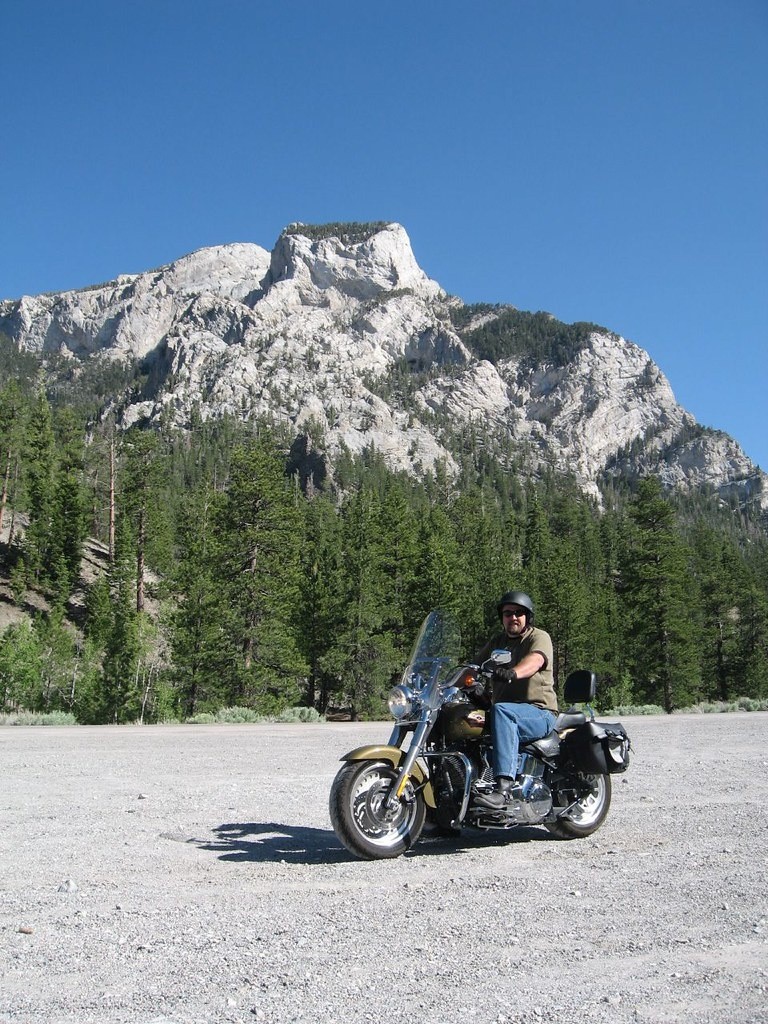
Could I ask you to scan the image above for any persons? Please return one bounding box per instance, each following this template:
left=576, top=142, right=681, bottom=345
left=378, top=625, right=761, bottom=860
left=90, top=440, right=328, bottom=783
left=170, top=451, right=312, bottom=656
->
left=472, top=591, right=559, bottom=809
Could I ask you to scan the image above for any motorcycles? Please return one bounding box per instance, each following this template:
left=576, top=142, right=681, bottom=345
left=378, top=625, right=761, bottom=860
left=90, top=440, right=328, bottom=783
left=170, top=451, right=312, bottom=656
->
left=329, top=608, right=631, bottom=859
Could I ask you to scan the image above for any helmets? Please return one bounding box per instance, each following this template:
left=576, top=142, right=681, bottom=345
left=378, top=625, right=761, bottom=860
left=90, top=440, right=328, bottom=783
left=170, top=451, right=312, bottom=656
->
left=497, top=591, right=533, bottom=613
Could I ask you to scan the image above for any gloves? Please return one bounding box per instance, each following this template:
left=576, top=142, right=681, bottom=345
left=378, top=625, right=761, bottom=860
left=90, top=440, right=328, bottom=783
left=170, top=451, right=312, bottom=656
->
left=494, top=668, right=517, bottom=682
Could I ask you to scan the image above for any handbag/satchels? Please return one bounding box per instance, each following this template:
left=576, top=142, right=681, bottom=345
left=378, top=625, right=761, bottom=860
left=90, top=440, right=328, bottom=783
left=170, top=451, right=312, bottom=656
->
left=566, top=721, right=630, bottom=776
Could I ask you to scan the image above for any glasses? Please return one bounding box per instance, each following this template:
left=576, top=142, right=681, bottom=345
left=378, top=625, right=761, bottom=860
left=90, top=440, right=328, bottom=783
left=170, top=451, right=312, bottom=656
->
left=501, top=610, right=529, bottom=617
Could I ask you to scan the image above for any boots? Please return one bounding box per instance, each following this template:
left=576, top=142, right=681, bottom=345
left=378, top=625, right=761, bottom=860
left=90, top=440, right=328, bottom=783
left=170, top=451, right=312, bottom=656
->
left=472, top=777, right=513, bottom=808
